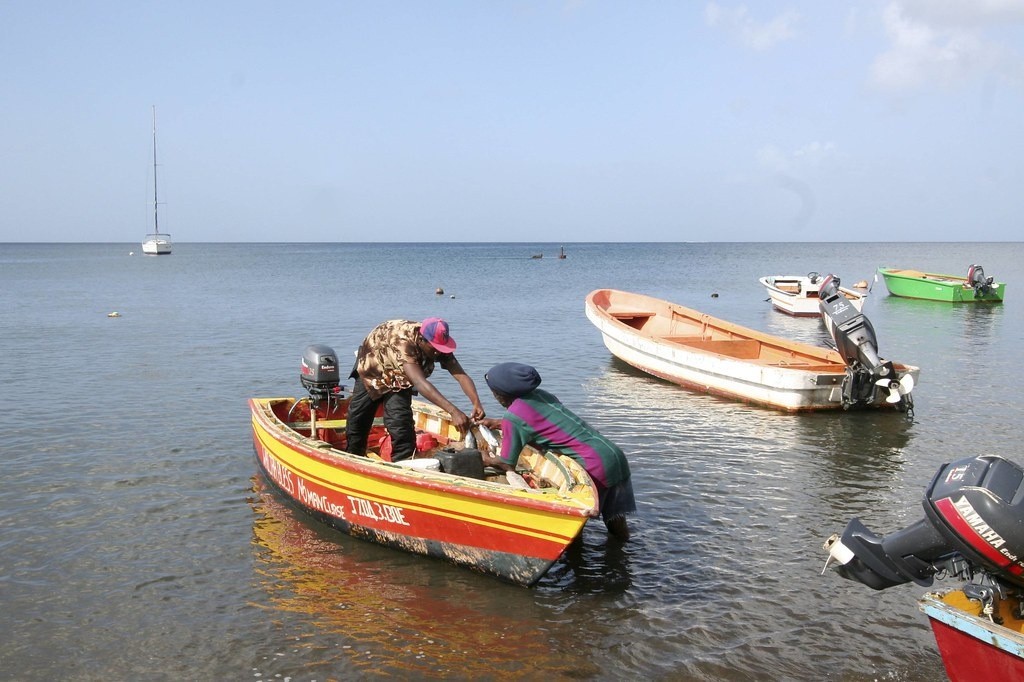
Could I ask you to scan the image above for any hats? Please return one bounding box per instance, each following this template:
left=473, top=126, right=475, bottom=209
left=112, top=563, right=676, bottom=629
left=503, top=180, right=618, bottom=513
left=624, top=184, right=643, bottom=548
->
left=420, top=317, right=457, bottom=355
left=485, top=362, right=542, bottom=394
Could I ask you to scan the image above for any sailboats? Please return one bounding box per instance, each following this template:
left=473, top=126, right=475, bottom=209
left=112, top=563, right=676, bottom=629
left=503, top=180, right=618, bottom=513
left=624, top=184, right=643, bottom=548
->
left=141, top=104, right=173, bottom=256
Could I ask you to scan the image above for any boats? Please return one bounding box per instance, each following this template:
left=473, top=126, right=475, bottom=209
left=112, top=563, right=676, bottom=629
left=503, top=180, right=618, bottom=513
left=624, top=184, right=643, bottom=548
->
left=877, top=266, right=1006, bottom=301
left=584, top=288, right=921, bottom=416
left=824, top=452, right=1024, bottom=680
left=244, top=344, right=604, bottom=587
left=761, top=274, right=868, bottom=318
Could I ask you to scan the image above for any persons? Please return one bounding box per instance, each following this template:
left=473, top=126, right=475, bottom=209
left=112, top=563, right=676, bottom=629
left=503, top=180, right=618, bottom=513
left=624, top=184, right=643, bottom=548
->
left=474, top=362, right=637, bottom=549
left=344, top=317, right=485, bottom=462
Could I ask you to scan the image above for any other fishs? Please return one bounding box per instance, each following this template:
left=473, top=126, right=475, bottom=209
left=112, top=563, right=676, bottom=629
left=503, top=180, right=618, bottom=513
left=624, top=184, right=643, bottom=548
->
left=472, top=417, right=501, bottom=447
left=463, top=426, right=478, bottom=449
